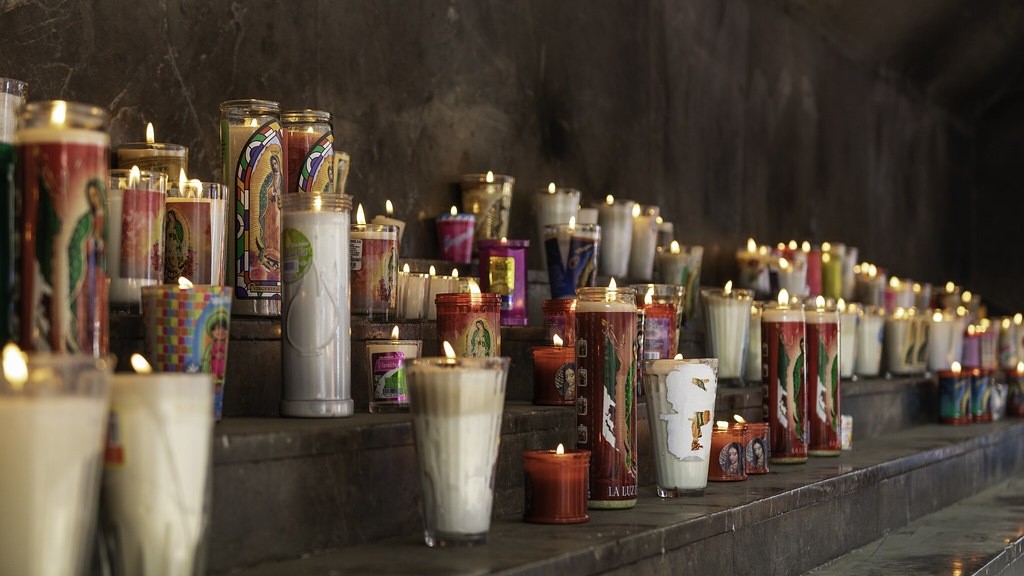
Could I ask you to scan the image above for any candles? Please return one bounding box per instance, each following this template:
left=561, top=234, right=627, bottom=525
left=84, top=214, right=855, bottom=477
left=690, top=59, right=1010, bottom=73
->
left=479, top=236, right=531, bottom=325
left=220, top=99, right=283, bottom=317
left=108, top=165, right=169, bottom=316
left=462, top=171, right=516, bottom=256
left=0, top=76, right=30, bottom=349
left=364, top=325, right=423, bottom=412
left=165, top=167, right=229, bottom=285
left=397, top=262, right=479, bottom=321
left=15, top=98, right=114, bottom=357
left=116, top=121, right=191, bottom=182
left=434, top=205, right=477, bottom=264
left=280, top=106, right=335, bottom=195
left=402, top=340, right=513, bottom=547
left=434, top=278, right=503, bottom=359
left=140, top=276, right=234, bottom=424
left=0, top=341, right=117, bottom=576
left=524, top=180, right=1024, bottom=526
left=349, top=203, right=398, bottom=323
left=279, top=191, right=354, bottom=418
left=92, top=351, right=217, bottom=576
